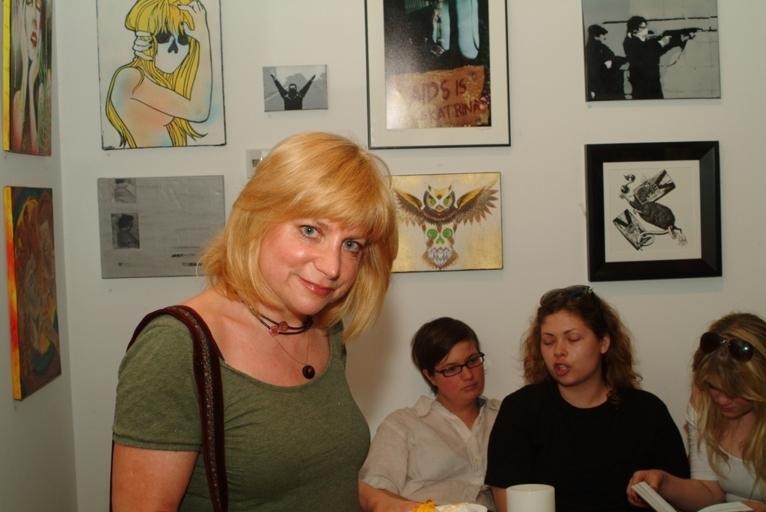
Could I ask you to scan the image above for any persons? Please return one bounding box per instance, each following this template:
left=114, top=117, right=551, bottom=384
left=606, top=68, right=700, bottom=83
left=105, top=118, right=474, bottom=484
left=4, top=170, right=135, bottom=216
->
left=108, top=132, right=398, bottom=512
left=626, top=312, right=765, bottom=512
left=622, top=14, right=697, bottom=98
left=117, top=214, right=137, bottom=248
left=269, top=73, right=314, bottom=109
left=13, top=1, right=50, bottom=156
left=114, top=179, right=135, bottom=203
left=484, top=284, right=691, bottom=511
left=585, top=24, right=628, bottom=101
left=358, top=317, right=501, bottom=512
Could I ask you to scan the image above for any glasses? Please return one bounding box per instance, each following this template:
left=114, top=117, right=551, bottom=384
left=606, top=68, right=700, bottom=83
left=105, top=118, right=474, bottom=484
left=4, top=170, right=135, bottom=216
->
left=700, top=331, right=753, bottom=362
left=540, top=286, right=601, bottom=305
left=434, top=352, right=485, bottom=377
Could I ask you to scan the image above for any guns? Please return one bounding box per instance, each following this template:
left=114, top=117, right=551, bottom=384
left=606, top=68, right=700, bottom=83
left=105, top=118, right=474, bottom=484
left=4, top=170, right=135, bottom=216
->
left=645, top=26, right=718, bottom=62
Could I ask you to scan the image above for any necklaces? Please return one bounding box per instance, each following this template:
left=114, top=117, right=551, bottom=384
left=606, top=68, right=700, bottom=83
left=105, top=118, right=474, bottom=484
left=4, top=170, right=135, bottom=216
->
left=247, top=302, right=315, bottom=380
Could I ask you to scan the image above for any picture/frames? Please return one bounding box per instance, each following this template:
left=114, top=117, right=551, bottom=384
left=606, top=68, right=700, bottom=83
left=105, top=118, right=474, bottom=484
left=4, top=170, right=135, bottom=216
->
left=584, top=140, right=722, bottom=282
left=365, top=0, right=511, bottom=150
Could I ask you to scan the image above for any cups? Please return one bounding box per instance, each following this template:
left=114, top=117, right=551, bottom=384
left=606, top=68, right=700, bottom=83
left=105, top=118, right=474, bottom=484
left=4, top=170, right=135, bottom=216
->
left=435, top=504, right=488, bottom=512
left=506, top=483, right=557, bottom=512
